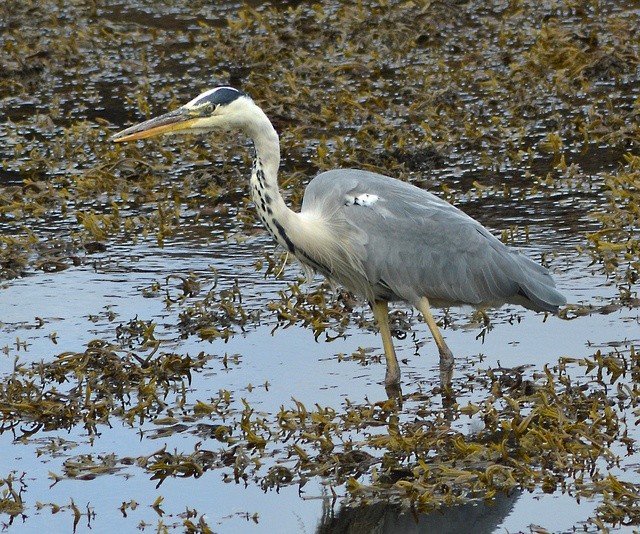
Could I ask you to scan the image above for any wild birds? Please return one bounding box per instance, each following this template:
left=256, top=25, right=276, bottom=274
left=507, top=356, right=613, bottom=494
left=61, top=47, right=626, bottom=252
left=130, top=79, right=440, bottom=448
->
left=107, top=86, right=567, bottom=387
